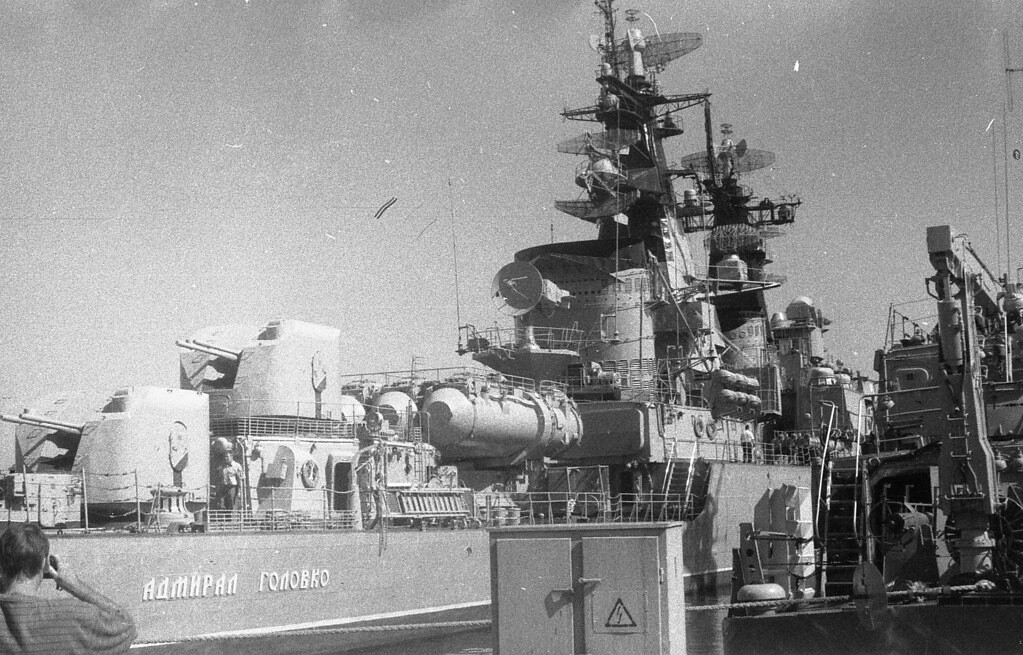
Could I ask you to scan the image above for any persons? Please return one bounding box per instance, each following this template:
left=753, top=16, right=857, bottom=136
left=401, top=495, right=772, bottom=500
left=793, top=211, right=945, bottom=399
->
left=741, top=425, right=756, bottom=463
left=0, top=523, right=138, bottom=655
left=219, top=451, right=242, bottom=522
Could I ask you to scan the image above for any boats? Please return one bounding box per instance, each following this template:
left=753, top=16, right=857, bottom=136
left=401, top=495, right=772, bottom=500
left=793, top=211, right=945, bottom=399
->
left=722, top=226, right=1023, bottom=655
left=0, top=0, right=875, bottom=651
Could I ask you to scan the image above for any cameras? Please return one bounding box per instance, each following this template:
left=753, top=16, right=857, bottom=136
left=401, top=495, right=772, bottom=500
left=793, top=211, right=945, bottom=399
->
left=43, top=554, right=57, bottom=579
